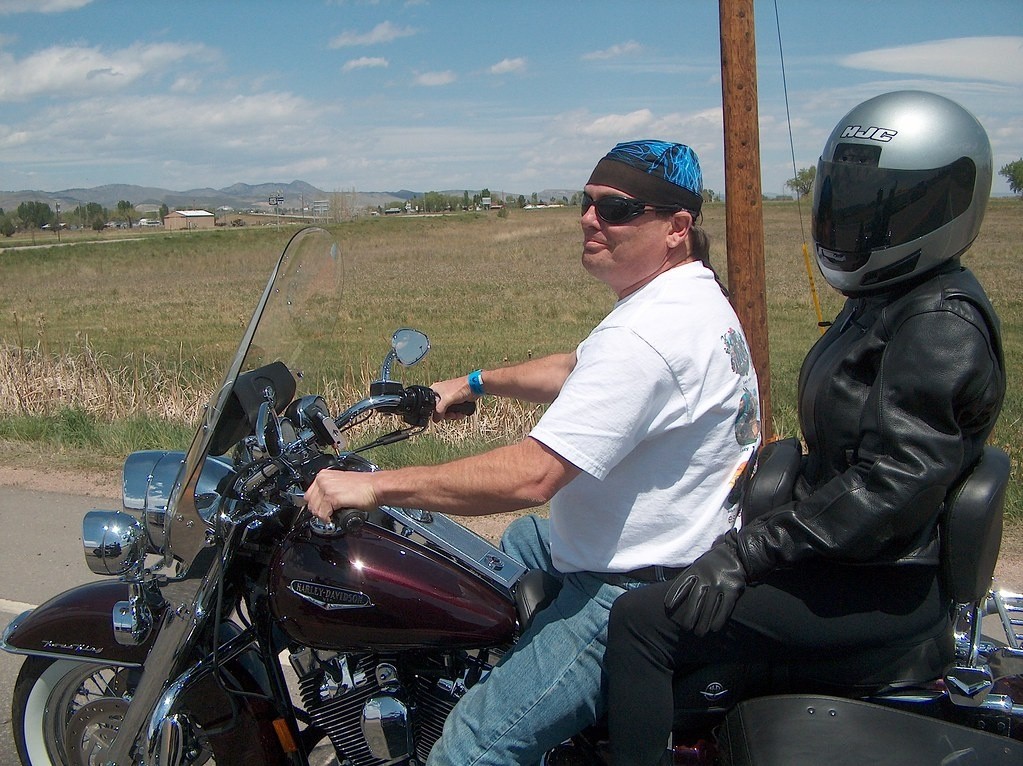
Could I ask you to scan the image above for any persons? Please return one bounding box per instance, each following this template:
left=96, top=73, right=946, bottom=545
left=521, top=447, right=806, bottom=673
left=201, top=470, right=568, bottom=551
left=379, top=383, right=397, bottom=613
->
left=302, top=139, right=763, bottom=766
left=600, top=90, right=1008, bottom=766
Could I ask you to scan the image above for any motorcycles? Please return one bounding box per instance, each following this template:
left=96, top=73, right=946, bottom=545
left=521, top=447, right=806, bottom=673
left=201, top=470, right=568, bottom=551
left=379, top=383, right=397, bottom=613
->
left=0, top=223, right=1023, bottom=766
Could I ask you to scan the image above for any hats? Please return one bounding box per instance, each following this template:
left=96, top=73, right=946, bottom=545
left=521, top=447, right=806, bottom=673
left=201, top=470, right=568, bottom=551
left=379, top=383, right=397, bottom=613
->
left=586, top=139, right=704, bottom=220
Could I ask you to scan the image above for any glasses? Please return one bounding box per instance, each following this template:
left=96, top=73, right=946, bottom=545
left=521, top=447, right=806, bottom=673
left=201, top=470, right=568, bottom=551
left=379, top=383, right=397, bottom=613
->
left=580, top=192, right=686, bottom=223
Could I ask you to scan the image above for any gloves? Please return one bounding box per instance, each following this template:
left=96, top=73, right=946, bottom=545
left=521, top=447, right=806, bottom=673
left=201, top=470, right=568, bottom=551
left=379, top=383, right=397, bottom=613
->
left=664, top=528, right=747, bottom=635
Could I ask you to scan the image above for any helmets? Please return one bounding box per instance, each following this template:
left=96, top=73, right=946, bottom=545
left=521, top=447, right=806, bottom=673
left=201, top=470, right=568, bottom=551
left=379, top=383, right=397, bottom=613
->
left=810, top=90, right=992, bottom=297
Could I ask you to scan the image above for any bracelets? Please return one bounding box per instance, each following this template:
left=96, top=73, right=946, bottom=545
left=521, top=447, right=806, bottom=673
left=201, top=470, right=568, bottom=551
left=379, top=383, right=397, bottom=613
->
left=468, top=369, right=485, bottom=396
